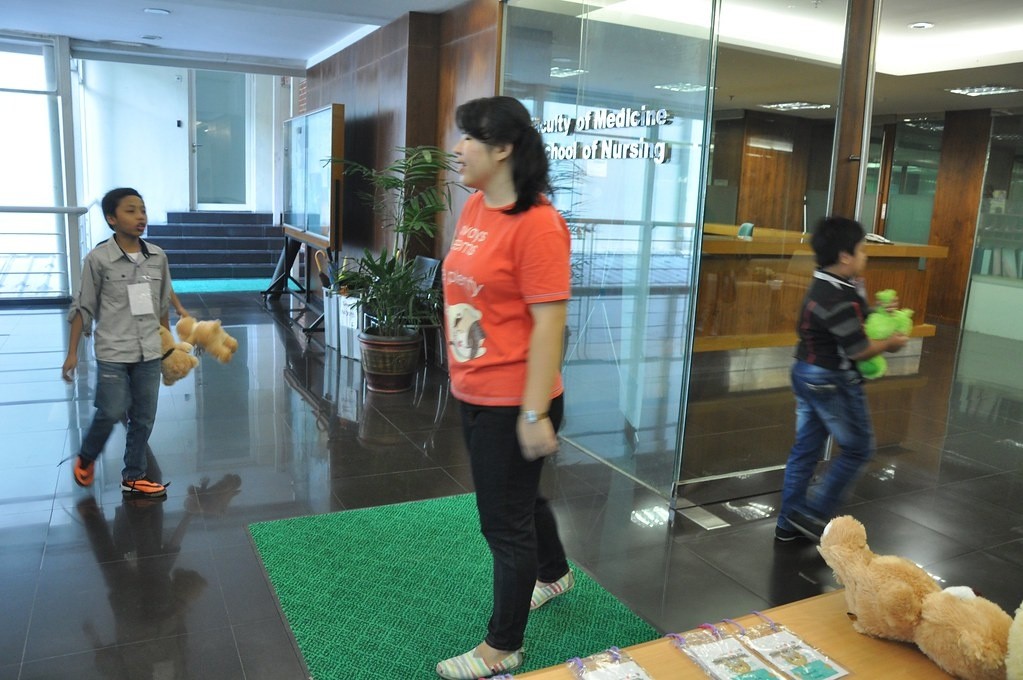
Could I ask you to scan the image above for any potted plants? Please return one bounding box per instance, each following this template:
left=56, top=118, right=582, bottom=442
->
left=320, top=144, right=473, bottom=392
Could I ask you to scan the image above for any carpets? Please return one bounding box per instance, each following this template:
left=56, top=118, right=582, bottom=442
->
left=249, top=492, right=664, bottom=680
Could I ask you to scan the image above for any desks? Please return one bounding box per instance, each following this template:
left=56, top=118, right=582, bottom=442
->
left=489, top=588, right=958, bottom=680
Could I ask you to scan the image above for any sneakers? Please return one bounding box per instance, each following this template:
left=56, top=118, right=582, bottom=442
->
left=121, top=477, right=166, bottom=497
left=75, top=455, right=94, bottom=486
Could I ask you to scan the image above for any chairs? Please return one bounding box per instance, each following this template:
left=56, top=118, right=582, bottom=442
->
left=362, top=255, right=444, bottom=365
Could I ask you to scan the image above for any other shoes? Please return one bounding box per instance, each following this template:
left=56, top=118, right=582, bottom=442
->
left=774, top=526, right=807, bottom=540
left=529, top=568, right=575, bottom=610
left=786, top=511, right=824, bottom=542
left=437, top=646, right=525, bottom=680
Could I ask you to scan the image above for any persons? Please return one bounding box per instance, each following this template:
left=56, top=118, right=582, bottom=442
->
left=61, top=187, right=189, bottom=499
left=772, top=215, right=909, bottom=541
left=435, top=95, right=580, bottom=680
left=78, top=496, right=195, bottom=680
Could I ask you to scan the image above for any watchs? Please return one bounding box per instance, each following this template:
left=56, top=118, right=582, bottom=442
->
left=520, top=409, right=548, bottom=423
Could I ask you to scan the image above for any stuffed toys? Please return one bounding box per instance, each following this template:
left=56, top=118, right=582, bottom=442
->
left=184, top=474, right=243, bottom=515
left=815, top=514, right=1023, bottom=680
left=173, top=566, right=207, bottom=601
left=158, top=323, right=199, bottom=387
left=857, top=289, right=914, bottom=378
left=175, top=317, right=238, bottom=364
